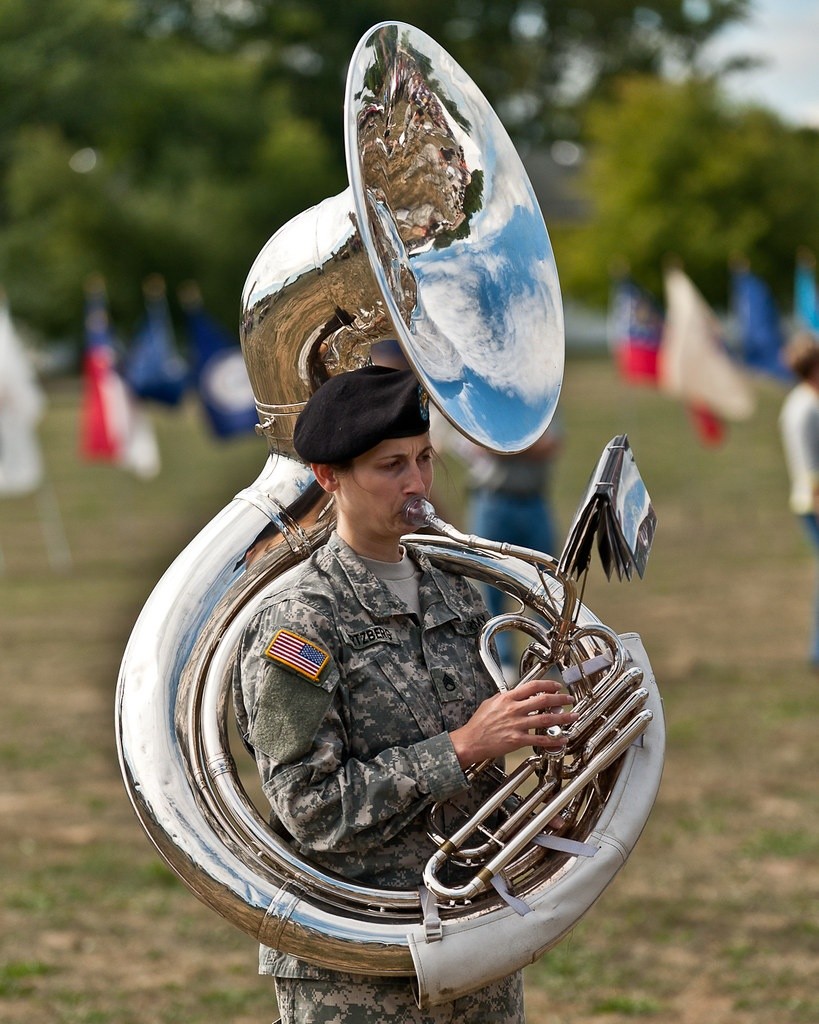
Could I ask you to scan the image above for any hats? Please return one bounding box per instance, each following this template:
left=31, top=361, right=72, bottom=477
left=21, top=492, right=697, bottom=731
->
left=294, top=365, right=430, bottom=466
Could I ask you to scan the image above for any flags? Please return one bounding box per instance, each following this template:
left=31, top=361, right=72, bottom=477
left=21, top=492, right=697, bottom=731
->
left=0, top=283, right=229, bottom=498
left=609, top=263, right=819, bottom=444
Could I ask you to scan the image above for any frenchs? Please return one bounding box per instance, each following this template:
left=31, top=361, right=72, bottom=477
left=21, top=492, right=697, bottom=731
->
left=116, top=16, right=655, bottom=977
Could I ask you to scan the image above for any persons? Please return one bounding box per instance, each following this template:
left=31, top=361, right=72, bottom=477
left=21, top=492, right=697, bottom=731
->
left=459, top=410, right=562, bottom=685
left=234, top=364, right=580, bottom=1024
left=780, top=329, right=818, bottom=667
